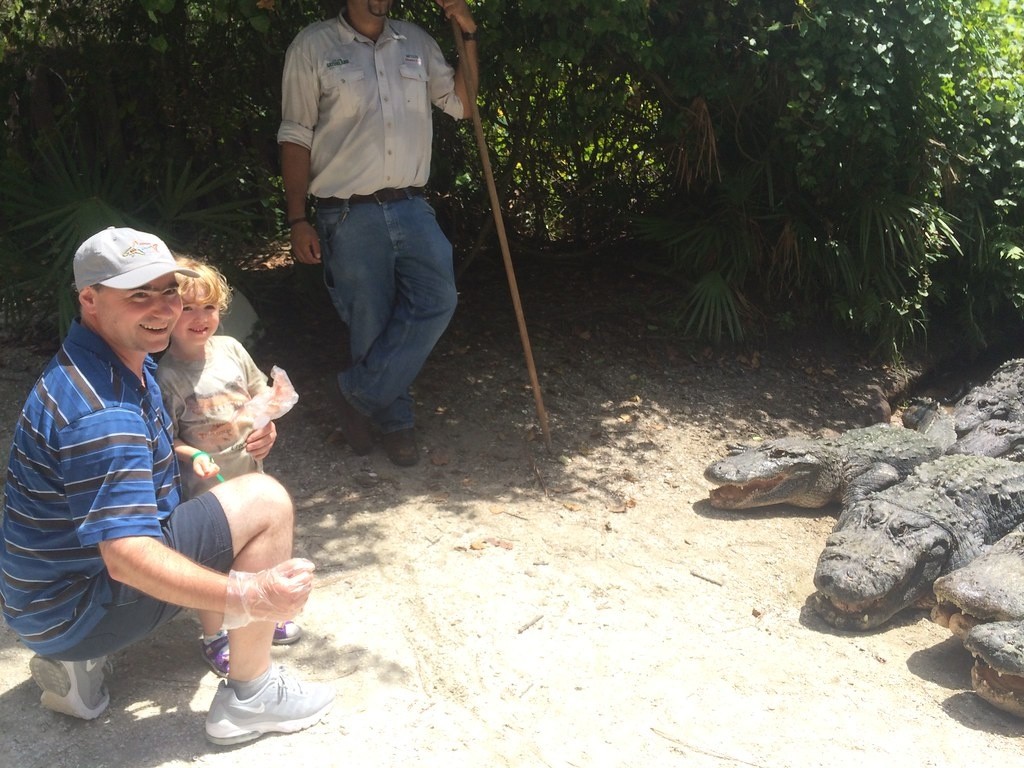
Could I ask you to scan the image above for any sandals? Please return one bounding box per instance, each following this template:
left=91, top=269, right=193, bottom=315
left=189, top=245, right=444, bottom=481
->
left=201, top=636, right=230, bottom=678
left=273, top=621, right=303, bottom=643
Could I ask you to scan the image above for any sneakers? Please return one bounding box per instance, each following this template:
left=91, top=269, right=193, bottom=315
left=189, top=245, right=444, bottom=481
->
left=205, top=664, right=336, bottom=745
left=29, top=653, right=113, bottom=721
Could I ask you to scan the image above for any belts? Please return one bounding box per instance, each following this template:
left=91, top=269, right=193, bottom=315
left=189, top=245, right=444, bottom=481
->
left=319, top=186, right=421, bottom=208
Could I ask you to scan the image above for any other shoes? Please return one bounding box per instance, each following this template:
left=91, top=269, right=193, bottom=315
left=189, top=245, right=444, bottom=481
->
left=384, top=428, right=420, bottom=466
left=320, top=374, right=375, bottom=455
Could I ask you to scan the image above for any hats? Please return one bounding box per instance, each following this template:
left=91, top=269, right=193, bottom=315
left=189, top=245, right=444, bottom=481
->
left=74, top=226, right=201, bottom=293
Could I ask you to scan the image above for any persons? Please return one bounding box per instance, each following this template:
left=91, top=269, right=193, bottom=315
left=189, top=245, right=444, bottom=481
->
left=277, top=0, right=479, bottom=467
left=0, top=226, right=337, bottom=745
left=154, top=258, right=302, bottom=678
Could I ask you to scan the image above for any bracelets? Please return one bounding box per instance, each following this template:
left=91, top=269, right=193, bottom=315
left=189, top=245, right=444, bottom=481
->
left=190, top=451, right=224, bottom=483
left=289, top=217, right=308, bottom=228
left=463, top=32, right=477, bottom=40
